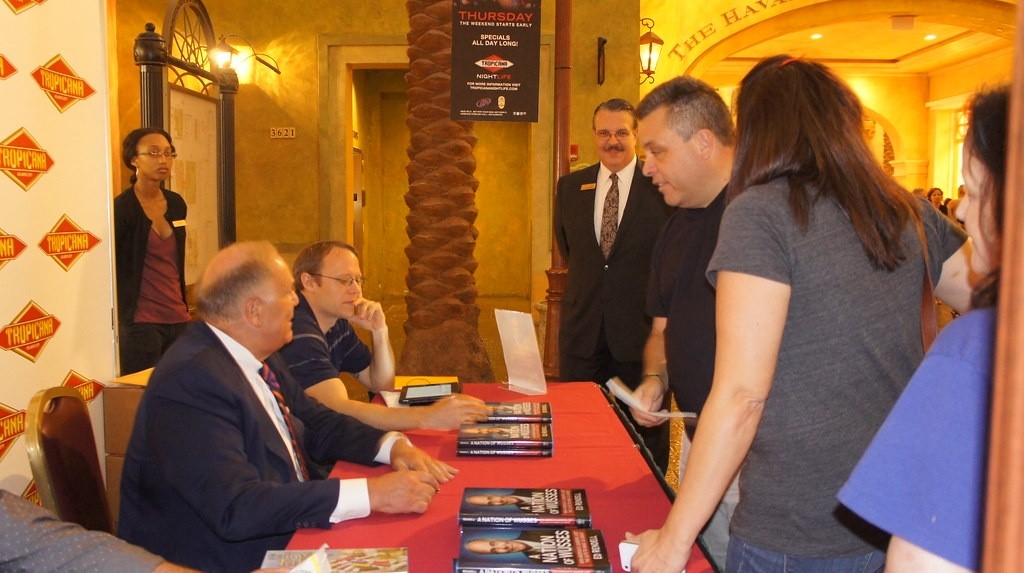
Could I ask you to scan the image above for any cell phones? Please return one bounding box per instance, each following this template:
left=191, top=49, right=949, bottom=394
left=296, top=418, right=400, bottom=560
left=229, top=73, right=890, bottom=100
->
left=619, top=540, right=686, bottom=573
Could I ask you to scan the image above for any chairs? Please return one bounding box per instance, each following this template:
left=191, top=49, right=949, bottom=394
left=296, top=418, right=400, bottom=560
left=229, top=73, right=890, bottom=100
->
left=23, top=386, right=113, bottom=533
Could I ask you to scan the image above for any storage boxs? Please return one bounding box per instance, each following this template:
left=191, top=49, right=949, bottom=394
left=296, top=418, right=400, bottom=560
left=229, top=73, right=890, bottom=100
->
left=102, top=366, right=155, bottom=536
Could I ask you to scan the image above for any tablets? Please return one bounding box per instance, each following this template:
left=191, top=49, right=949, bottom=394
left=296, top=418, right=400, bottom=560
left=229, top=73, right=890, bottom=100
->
left=397, top=382, right=461, bottom=404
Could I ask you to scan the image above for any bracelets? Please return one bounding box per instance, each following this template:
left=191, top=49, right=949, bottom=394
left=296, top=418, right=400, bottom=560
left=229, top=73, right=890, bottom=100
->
left=640, top=373, right=666, bottom=400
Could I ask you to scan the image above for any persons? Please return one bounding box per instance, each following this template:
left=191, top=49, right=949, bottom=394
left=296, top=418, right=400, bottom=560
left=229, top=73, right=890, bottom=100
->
left=115, top=238, right=493, bottom=573
left=553, top=98, right=679, bottom=450
left=0, top=489, right=288, bottom=573
left=837, top=84, right=1009, bottom=573
left=626, top=53, right=970, bottom=573
left=114, top=128, right=195, bottom=376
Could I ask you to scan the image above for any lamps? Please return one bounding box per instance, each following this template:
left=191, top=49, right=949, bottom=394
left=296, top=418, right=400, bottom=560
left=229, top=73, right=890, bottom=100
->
left=207, top=32, right=281, bottom=74
left=639, top=16, right=664, bottom=83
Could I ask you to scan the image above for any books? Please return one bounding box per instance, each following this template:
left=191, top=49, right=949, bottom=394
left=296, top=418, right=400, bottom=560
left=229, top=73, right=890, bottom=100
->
left=455, top=487, right=611, bottom=573
left=456, top=401, right=551, bottom=458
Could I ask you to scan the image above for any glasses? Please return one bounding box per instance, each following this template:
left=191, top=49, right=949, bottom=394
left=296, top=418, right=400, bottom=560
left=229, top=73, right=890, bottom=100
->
left=135, top=148, right=177, bottom=160
left=592, top=129, right=635, bottom=141
left=308, top=273, right=367, bottom=289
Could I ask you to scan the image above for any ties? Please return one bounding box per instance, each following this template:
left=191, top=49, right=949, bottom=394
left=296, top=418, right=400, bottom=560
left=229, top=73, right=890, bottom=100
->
left=259, top=363, right=310, bottom=486
left=600, top=173, right=620, bottom=261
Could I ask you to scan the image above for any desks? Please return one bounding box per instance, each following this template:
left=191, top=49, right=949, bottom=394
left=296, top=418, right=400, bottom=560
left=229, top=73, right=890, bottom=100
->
left=282, top=380, right=722, bottom=573
